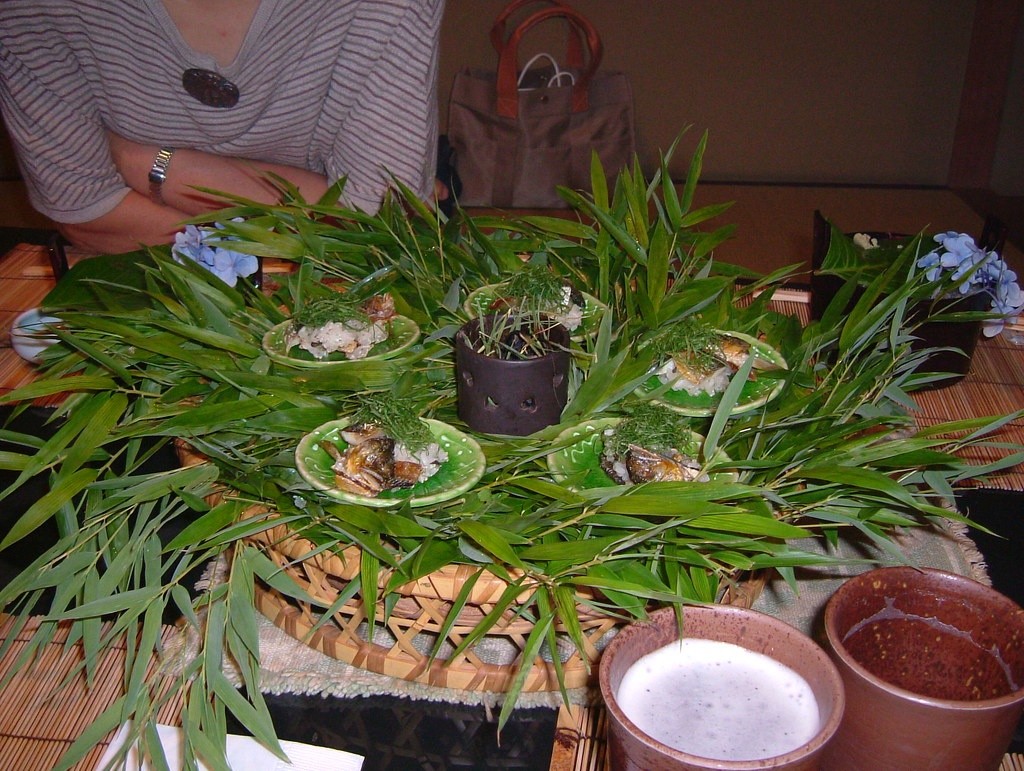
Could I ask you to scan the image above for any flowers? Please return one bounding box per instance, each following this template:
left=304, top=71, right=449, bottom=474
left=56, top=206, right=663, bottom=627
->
left=916, top=228, right=1024, bottom=337
left=172, top=213, right=259, bottom=289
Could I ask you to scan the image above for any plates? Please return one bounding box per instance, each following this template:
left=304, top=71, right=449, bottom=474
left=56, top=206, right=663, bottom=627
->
left=462, top=282, right=612, bottom=344
left=632, top=329, right=789, bottom=417
left=547, top=417, right=740, bottom=495
left=262, top=312, right=422, bottom=371
left=294, top=416, right=486, bottom=508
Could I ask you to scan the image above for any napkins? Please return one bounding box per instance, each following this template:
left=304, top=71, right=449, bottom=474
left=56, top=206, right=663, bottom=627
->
left=95, top=719, right=364, bottom=771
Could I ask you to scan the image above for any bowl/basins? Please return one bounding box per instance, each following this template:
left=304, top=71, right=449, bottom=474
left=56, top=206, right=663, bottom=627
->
left=11, top=306, right=69, bottom=366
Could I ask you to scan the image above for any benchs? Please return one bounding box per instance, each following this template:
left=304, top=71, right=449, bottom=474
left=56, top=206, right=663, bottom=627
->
left=0, top=0, right=1024, bottom=287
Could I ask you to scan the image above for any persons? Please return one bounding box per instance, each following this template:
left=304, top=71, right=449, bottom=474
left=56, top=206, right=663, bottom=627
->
left=0, top=0, right=447, bottom=255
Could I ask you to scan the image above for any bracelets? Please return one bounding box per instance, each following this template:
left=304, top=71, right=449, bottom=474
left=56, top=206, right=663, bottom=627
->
left=148, top=146, right=176, bottom=207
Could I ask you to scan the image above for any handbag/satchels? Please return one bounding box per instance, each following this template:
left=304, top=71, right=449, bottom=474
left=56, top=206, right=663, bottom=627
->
left=446, top=0, right=637, bottom=210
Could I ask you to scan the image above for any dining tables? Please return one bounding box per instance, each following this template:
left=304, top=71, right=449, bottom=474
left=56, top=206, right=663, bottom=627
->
left=0, top=237, right=1024, bottom=771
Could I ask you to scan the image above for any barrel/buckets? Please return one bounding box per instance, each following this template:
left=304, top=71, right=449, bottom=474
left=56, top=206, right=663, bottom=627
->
left=821, top=566, right=1024, bottom=771
left=598, top=602, right=847, bottom=771
